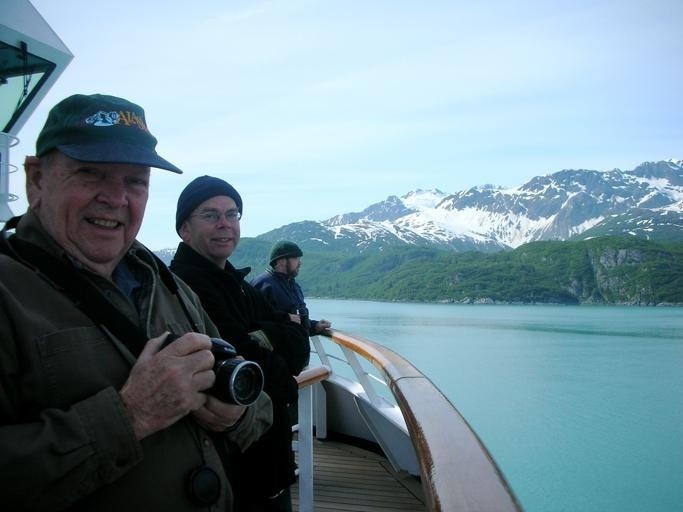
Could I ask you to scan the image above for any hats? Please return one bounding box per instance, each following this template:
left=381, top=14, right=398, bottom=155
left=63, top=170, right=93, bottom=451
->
left=176, top=176, right=242, bottom=239
left=36, top=93, right=183, bottom=175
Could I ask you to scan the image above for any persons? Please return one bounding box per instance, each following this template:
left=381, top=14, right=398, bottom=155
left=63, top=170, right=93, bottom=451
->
left=249, top=238, right=333, bottom=483
left=0, top=93, right=276, bottom=510
left=168, top=174, right=310, bottom=511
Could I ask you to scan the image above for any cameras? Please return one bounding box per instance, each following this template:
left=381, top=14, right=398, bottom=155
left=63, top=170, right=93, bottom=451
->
left=158, top=333, right=265, bottom=407
left=291, top=303, right=308, bottom=319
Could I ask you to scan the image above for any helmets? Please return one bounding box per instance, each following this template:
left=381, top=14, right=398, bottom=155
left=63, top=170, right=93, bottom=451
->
left=191, top=209, right=244, bottom=223
left=269, top=240, right=303, bottom=266
